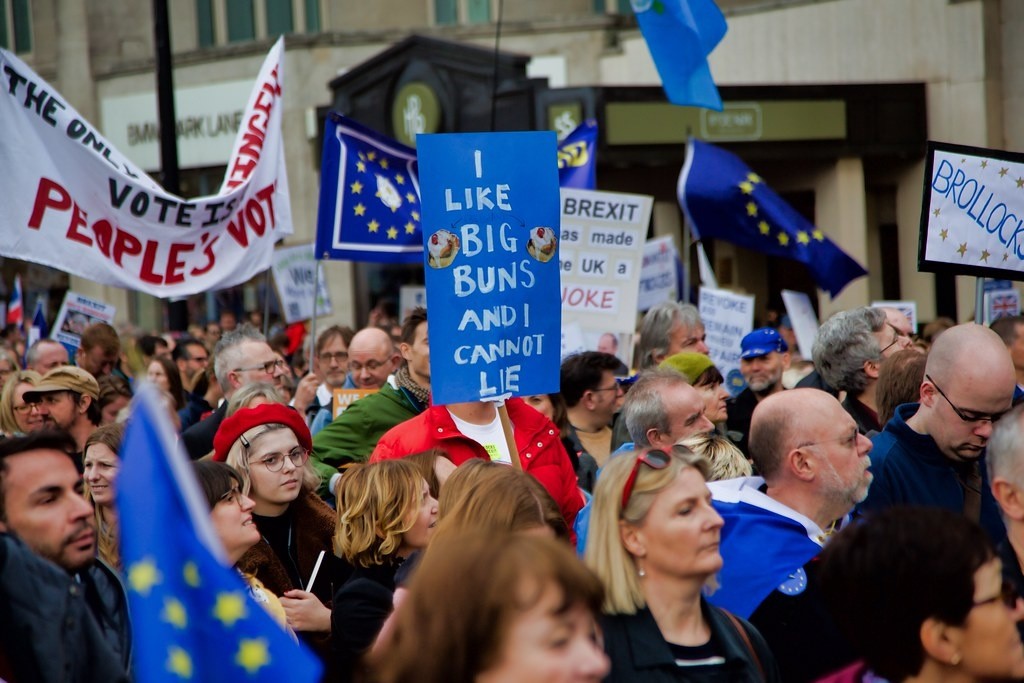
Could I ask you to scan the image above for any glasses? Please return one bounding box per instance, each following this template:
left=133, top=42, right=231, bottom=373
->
left=618, top=444, right=694, bottom=520
left=352, top=356, right=391, bottom=369
left=215, top=488, right=244, bottom=508
left=12, top=404, right=39, bottom=415
left=593, top=384, right=619, bottom=393
left=1, top=370, right=12, bottom=376
left=233, top=360, right=283, bottom=374
left=971, top=583, right=1017, bottom=612
left=191, top=357, right=204, bottom=363
left=798, top=429, right=859, bottom=450
left=927, top=374, right=1006, bottom=423
left=250, top=446, right=310, bottom=473
left=879, top=333, right=898, bottom=354
left=320, top=351, right=348, bottom=362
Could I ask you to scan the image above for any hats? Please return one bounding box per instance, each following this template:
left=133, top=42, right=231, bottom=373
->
left=22, top=365, right=100, bottom=404
left=659, top=350, right=715, bottom=386
left=741, top=328, right=789, bottom=358
left=212, top=403, right=312, bottom=462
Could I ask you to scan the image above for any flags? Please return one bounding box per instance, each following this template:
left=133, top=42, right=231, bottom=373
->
left=678, top=138, right=868, bottom=298
left=630, top=0, right=728, bottom=112
left=114, top=375, right=322, bottom=683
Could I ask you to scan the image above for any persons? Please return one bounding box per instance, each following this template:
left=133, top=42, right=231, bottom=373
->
left=0, top=307, right=1023, bottom=683
